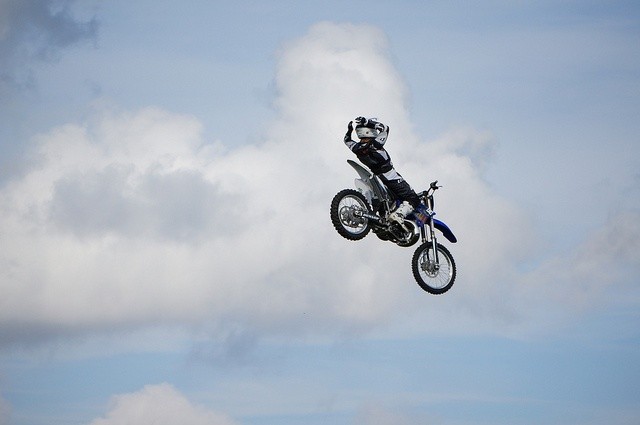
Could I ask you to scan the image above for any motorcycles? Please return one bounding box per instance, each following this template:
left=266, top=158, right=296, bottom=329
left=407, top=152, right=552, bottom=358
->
left=329, top=160, right=457, bottom=295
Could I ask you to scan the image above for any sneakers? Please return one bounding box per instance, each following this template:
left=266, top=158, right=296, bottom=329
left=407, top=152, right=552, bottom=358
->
left=388, top=211, right=409, bottom=235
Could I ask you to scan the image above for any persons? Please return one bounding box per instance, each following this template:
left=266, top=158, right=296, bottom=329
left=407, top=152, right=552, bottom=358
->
left=343, top=116, right=421, bottom=231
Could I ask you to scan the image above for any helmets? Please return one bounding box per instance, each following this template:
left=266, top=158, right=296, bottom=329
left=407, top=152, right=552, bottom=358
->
left=356, top=117, right=378, bottom=139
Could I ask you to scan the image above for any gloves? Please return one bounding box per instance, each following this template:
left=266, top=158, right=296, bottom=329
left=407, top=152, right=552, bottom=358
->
left=347, top=121, right=354, bottom=132
left=354, top=115, right=369, bottom=126
